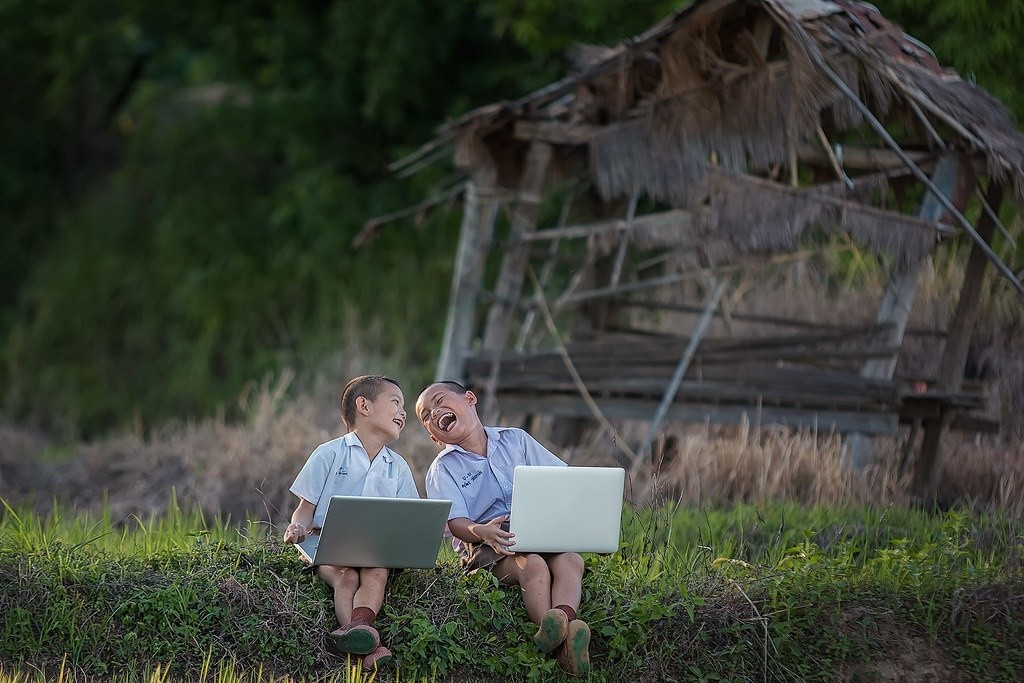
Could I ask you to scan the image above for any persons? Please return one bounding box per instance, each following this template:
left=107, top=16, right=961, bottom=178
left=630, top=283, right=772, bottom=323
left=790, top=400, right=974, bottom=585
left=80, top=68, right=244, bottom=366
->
left=281, top=375, right=424, bottom=666
left=416, top=382, right=593, bottom=676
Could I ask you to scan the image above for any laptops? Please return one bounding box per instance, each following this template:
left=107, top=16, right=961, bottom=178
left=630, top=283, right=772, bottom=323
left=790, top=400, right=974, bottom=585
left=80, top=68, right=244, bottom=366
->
left=292, top=495, right=452, bottom=569
left=501, top=465, right=626, bottom=556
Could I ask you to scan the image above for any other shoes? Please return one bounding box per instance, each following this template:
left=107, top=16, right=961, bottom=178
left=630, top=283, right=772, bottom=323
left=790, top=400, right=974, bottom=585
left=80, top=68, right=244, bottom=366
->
left=361, top=646, right=392, bottom=671
left=560, top=619, right=591, bottom=676
left=533, top=608, right=569, bottom=653
left=330, top=623, right=380, bottom=655
